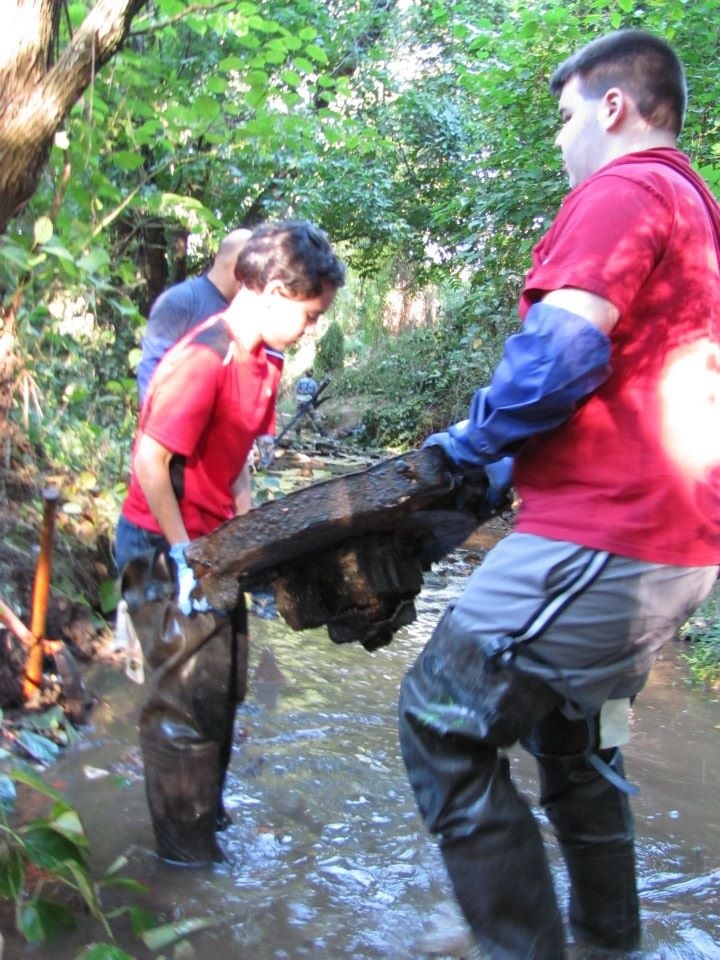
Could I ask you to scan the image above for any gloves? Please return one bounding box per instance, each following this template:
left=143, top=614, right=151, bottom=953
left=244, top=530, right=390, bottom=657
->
left=477, top=456, right=514, bottom=504
left=423, top=303, right=612, bottom=470
left=170, top=545, right=219, bottom=614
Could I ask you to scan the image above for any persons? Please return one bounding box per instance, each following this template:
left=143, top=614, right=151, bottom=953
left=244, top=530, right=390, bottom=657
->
left=294, top=368, right=328, bottom=437
left=115, top=222, right=344, bottom=863
left=396, top=30, right=720, bottom=959
left=138, top=229, right=253, bottom=406
